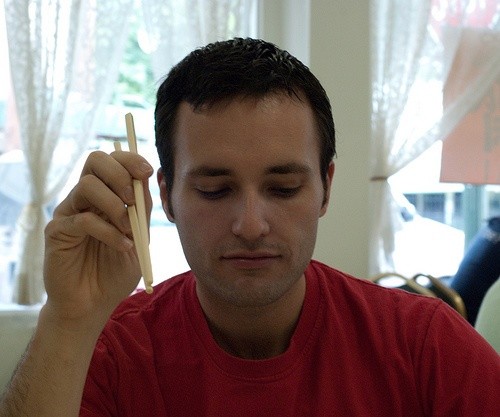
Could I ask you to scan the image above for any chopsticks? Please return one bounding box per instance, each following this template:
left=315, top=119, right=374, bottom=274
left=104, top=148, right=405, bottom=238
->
left=114, top=111, right=154, bottom=295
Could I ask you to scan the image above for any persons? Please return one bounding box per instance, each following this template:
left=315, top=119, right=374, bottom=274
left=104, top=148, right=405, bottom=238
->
left=449, top=216, right=500, bottom=326
left=1, top=37, right=500, bottom=417
